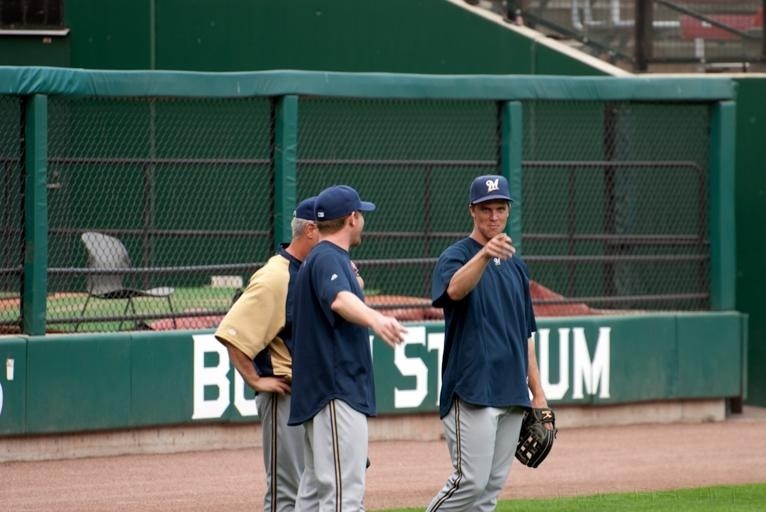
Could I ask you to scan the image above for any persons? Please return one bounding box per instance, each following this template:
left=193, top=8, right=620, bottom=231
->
left=424, top=174, right=551, bottom=512
left=213, top=195, right=370, bottom=511
left=287, top=185, right=409, bottom=512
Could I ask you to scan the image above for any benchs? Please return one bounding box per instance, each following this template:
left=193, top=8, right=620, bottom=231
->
left=681, top=7, right=765, bottom=73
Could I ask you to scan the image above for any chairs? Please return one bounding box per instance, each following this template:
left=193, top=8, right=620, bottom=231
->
left=69, top=231, right=178, bottom=332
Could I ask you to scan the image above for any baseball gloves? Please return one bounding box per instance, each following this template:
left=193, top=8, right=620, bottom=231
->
left=516, top=406, right=558, bottom=469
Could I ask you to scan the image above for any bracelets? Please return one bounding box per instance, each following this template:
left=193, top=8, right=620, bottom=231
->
left=353, top=269, right=361, bottom=277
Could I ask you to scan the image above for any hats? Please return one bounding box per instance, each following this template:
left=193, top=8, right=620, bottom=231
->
left=468, top=175, right=514, bottom=208
left=314, top=184, right=376, bottom=221
left=292, top=196, right=318, bottom=221
